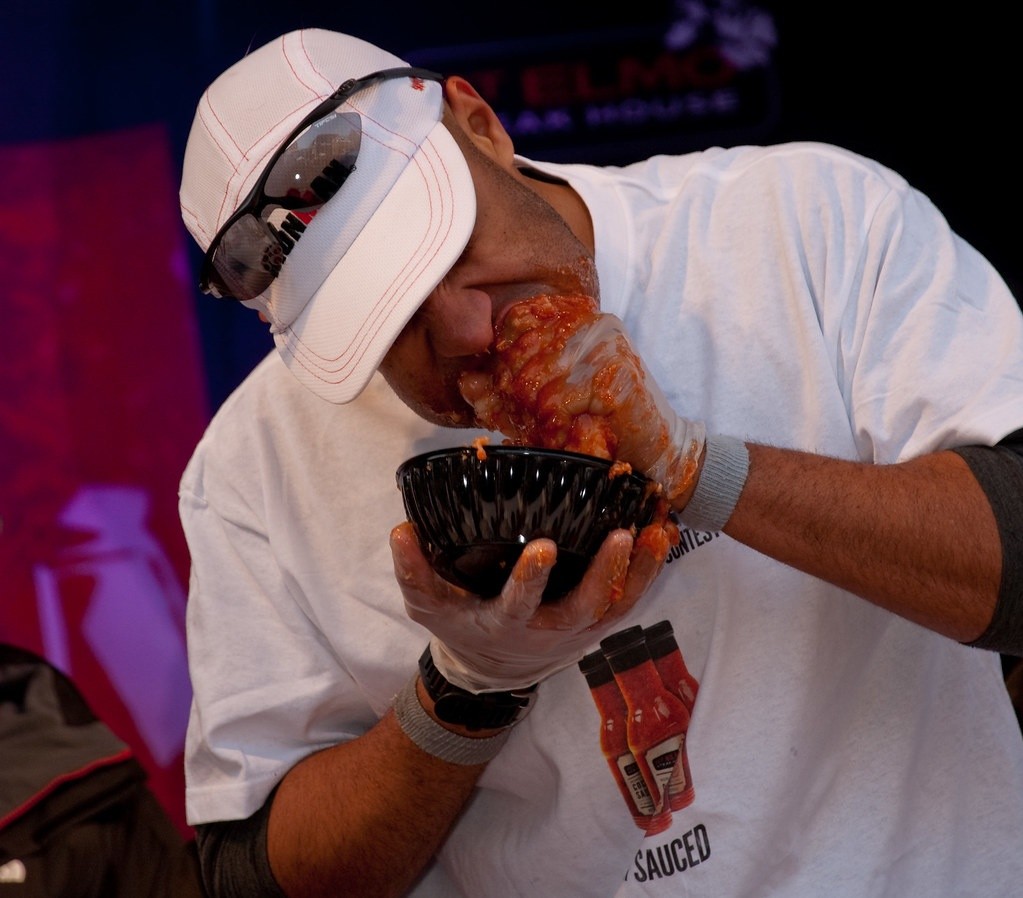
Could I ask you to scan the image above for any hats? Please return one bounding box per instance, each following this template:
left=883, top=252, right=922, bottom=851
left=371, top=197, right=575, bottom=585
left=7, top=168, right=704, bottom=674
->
left=178, top=26, right=475, bottom=404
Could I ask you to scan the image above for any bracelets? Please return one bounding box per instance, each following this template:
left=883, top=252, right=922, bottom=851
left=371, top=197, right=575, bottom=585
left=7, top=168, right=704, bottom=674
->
left=415, top=643, right=528, bottom=727
left=397, top=672, right=517, bottom=765
left=673, top=434, right=751, bottom=533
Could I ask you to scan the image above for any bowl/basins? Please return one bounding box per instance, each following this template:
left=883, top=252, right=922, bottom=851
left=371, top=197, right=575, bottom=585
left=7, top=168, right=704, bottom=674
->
left=396, top=444, right=658, bottom=603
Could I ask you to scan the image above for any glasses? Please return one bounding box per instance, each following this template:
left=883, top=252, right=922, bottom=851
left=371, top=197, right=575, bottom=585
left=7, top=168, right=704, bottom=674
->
left=199, top=68, right=445, bottom=301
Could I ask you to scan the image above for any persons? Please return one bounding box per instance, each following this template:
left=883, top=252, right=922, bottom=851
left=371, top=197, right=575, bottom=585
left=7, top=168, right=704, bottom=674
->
left=178, top=29, right=1023, bottom=898
left=0, top=640, right=199, bottom=898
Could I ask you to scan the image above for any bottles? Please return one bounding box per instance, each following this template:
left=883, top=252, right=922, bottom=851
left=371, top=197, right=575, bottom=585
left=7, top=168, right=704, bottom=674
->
left=578, top=620, right=699, bottom=837
